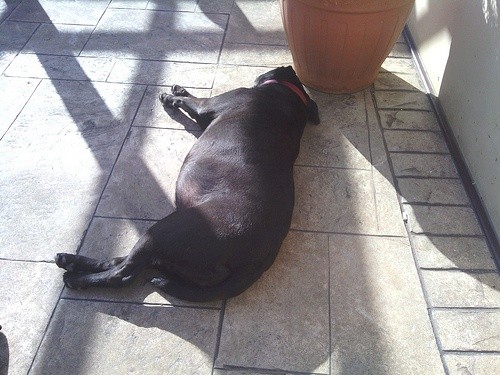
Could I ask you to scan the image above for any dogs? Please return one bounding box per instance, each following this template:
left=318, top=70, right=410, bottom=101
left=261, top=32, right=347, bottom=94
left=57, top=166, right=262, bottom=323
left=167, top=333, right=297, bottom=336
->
left=54, top=65, right=321, bottom=302
